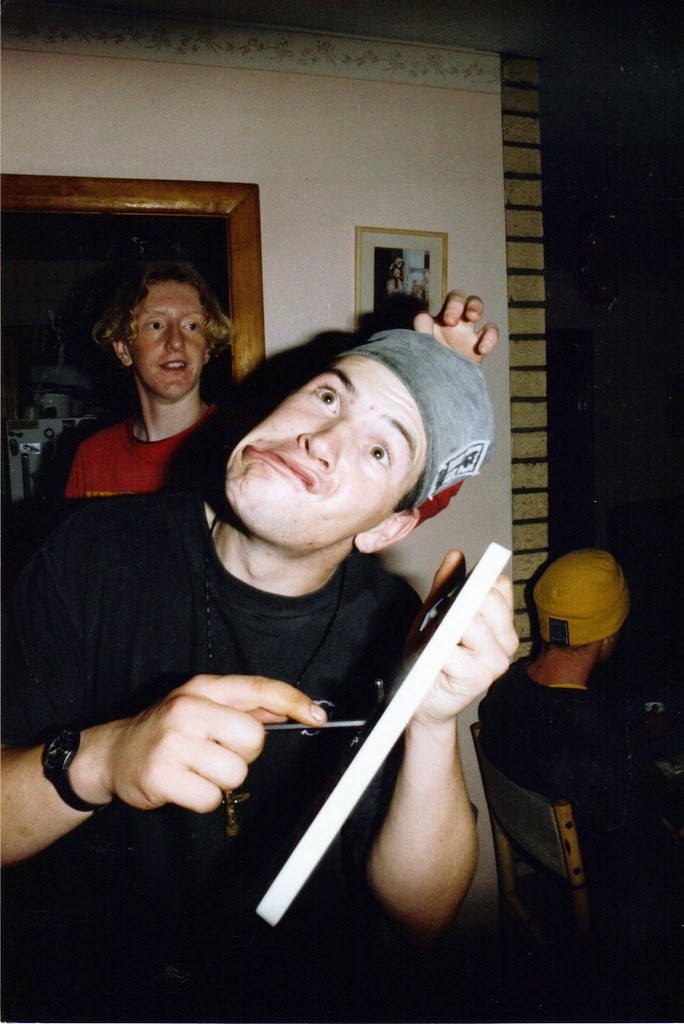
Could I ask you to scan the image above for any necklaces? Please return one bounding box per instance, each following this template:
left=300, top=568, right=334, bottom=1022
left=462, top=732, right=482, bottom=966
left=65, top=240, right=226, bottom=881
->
left=204, top=510, right=347, bottom=838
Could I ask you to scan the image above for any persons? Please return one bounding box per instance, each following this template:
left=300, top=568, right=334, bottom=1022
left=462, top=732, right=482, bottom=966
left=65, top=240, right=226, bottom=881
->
left=0, top=331, right=520, bottom=1024
left=477, top=546, right=684, bottom=1023
left=64, top=259, right=501, bottom=498
left=386, top=258, right=426, bottom=296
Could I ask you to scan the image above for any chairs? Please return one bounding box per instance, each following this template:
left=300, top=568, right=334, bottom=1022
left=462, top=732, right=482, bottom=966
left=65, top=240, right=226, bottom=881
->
left=472, top=723, right=684, bottom=1023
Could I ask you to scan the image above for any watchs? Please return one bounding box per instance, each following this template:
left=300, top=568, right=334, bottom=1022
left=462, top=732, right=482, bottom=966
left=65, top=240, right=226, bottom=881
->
left=41, top=726, right=112, bottom=812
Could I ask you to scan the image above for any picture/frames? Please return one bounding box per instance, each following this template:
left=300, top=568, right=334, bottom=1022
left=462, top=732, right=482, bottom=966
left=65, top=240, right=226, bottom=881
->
left=355, top=226, right=449, bottom=345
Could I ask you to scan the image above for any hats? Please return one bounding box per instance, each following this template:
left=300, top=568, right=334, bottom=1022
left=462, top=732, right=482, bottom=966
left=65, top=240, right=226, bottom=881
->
left=342, top=324, right=494, bottom=527
left=533, top=547, right=630, bottom=650
